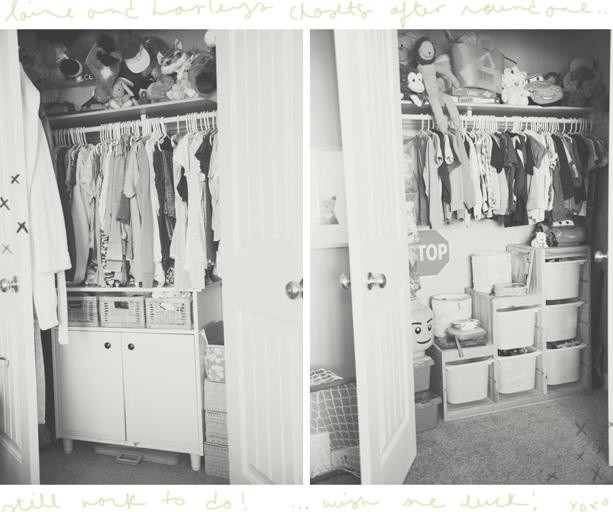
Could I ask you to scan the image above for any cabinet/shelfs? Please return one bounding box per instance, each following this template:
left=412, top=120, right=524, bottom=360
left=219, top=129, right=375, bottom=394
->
left=429, top=241, right=594, bottom=422
left=214, top=30, right=418, bottom=484
left=50, top=280, right=221, bottom=472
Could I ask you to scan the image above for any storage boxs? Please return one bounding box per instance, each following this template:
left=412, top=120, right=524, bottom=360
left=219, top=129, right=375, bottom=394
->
left=414, top=355, right=442, bottom=432
left=202, top=377, right=230, bottom=479
left=445, top=259, right=588, bottom=405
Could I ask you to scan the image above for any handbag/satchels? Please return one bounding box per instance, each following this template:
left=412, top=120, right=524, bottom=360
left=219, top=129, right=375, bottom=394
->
left=450, top=31, right=505, bottom=96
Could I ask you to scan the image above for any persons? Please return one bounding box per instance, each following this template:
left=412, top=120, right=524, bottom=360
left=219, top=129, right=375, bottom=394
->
left=398, top=39, right=604, bottom=135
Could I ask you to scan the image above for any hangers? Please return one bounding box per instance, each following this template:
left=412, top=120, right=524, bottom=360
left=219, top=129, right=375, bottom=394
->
left=403, top=113, right=605, bottom=149
left=50, top=108, right=219, bottom=162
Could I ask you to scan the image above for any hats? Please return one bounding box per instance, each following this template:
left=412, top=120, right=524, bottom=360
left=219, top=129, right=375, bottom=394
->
left=119, top=31, right=152, bottom=76
